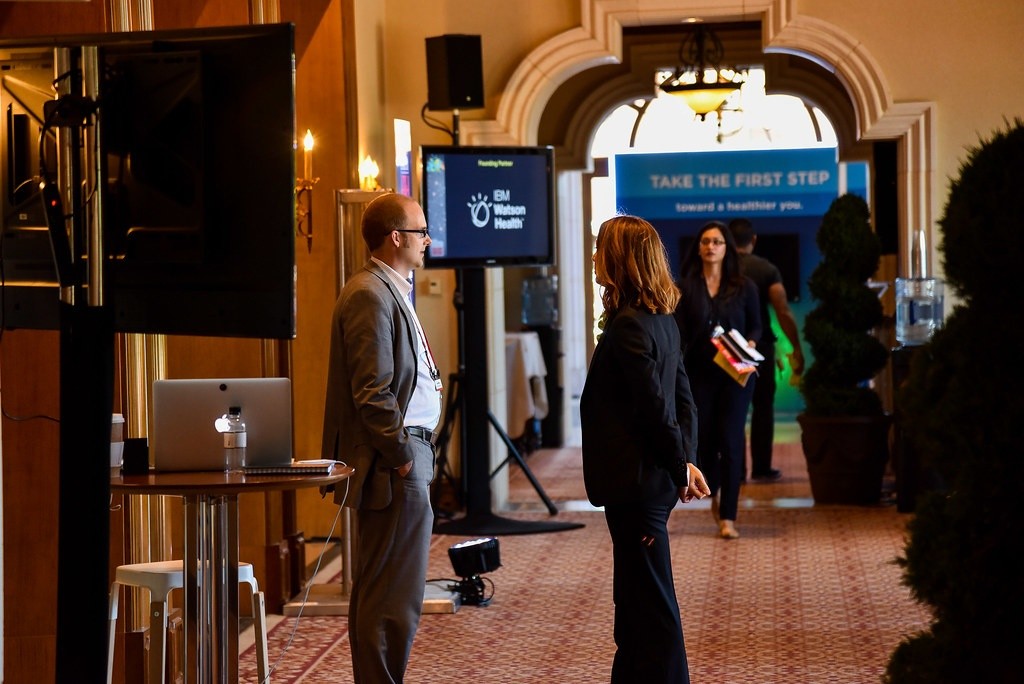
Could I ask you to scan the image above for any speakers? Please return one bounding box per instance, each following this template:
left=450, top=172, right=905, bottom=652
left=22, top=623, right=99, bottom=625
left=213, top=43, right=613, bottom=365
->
left=424, top=33, right=483, bottom=112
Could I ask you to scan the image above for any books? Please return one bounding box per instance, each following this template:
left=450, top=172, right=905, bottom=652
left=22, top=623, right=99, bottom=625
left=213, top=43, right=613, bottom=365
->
left=244, top=462, right=335, bottom=475
left=710, top=327, right=765, bottom=388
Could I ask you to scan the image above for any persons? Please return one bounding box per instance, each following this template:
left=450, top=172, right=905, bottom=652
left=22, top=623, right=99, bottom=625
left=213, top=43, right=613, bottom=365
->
left=579, top=215, right=712, bottom=684
left=672, top=220, right=763, bottom=539
left=319, top=193, right=444, bottom=684
left=722, top=217, right=805, bottom=483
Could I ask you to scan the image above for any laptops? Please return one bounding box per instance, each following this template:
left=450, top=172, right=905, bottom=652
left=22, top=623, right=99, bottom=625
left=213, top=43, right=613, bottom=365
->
left=153, top=378, right=293, bottom=475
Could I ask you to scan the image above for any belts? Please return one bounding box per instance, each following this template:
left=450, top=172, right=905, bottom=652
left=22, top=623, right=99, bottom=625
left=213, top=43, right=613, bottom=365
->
left=407, top=427, right=438, bottom=443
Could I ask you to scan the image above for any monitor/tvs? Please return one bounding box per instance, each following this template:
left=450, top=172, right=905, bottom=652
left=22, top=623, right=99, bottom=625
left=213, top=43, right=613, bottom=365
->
left=418, top=144, right=554, bottom=269
left=1, top=23, right=299, bottom=341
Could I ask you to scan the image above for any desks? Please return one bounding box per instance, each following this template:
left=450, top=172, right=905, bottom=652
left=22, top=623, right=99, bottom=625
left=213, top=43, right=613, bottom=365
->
left=111, top=463, right=356, bottom=684
left=505, top=332, right=548, bottom=465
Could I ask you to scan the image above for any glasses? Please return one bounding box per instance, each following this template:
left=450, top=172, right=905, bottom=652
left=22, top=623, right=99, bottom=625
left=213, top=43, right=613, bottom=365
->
left=700, top=240, right=726, bottom=246
left=385, top=228, right=428, bottom=238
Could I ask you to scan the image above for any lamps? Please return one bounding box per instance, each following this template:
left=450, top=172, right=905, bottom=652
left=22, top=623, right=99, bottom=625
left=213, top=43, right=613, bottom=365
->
left=660, top=31, right=745, bottom=121
left=447, top=537, right=504, bottom=606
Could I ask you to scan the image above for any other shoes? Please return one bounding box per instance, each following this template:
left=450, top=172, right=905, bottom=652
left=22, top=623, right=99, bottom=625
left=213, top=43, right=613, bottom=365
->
left=720, top=526, right=739, bottom=538
left=711, top=496, right=722, bottom=524
left=756, top=470, right=781, bottom=481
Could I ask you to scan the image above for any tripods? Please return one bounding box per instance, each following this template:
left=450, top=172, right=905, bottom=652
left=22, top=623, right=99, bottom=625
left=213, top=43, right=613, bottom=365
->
left=431, top=108, right=559, bottom=520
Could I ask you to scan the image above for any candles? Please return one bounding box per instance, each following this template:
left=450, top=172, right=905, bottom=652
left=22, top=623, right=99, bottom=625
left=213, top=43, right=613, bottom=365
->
left=361, top=153, right=380, bottom=190
left=303, top=128, right=315, bottom=180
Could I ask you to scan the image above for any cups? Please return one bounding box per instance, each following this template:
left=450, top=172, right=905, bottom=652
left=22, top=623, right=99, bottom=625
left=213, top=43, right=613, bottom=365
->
left=109, top=413, right=125, bottom=478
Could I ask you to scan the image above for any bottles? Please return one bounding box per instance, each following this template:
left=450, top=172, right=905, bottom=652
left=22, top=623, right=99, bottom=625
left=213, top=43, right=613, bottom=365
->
left=224, top=406, right=246, bottom=473
left=894, top=277, right=937, bottom=347
left=517, top=266, right=559, bottom=326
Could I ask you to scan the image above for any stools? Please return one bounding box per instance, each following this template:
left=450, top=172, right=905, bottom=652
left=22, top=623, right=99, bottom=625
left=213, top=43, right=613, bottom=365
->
left=107, top=559, right=270, bottom=684
